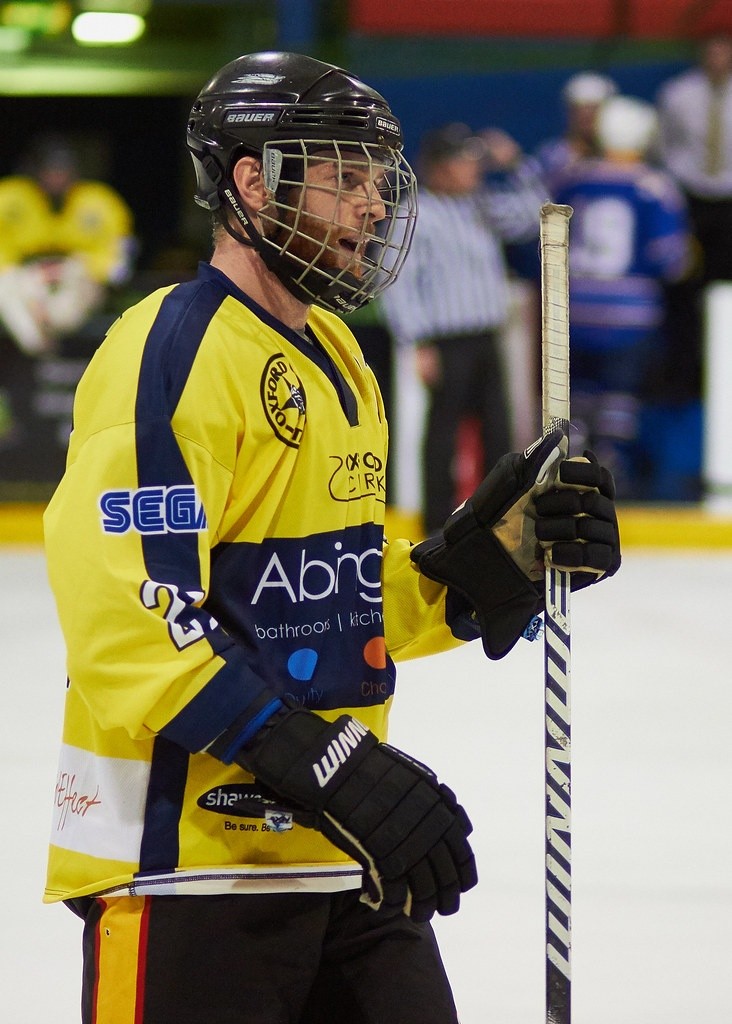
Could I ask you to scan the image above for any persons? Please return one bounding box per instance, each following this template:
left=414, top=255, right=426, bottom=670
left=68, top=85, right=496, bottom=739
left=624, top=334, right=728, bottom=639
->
left=38, top=49, right=621, bottom=1024
left=378, top=24, right=732, bottom=540
left=1, top=120, right=137, bottom=473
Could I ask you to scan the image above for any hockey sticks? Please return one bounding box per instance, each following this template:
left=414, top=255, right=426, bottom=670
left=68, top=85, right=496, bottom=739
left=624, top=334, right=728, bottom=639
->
left=534, top=198, right=579, bottom=1024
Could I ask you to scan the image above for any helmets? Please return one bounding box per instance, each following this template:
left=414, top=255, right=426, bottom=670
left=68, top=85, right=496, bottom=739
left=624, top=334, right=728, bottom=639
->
left=185, top=51, right=418, bottom=315
left=595, top=97, right=661, bottom=154
left=565, top=71, right=613, bottom=108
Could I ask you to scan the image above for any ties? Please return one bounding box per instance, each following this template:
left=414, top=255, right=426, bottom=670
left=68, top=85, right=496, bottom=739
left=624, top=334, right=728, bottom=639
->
left=706, top=80, right=722, bottom=176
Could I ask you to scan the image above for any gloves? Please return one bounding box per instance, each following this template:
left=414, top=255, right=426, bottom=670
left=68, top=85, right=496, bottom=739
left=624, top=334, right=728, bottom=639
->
left=208, top=688, right=478, bottom=923
left=409, top=428, right=622, bottom=661
